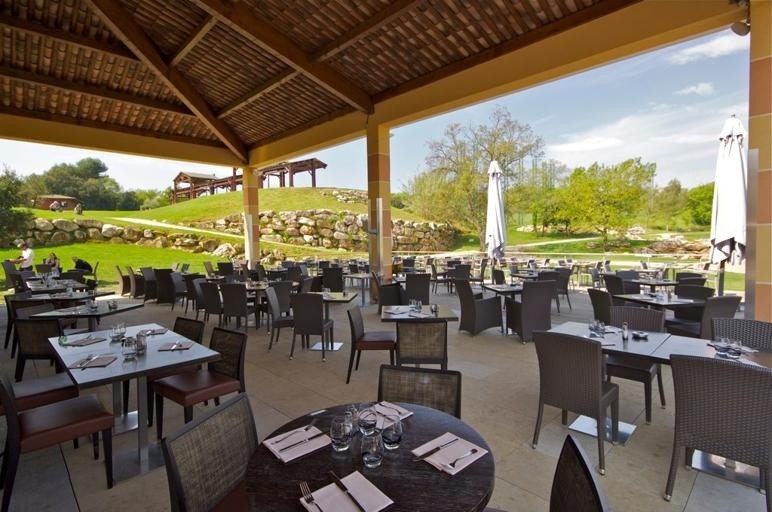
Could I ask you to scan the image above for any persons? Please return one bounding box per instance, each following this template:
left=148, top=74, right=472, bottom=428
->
left=47, top=252, right=59, bottom=270
left=9, top=244, right=33, bottom=272
left=73, top=256, right=92, bottom=272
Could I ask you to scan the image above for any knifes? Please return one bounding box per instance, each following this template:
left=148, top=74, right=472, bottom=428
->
left=80, top=354, right=100, bottom=368
left=170, top=340, right=179, bottom=350
left=328, top=470, right=367, bottom=512
left=279, top=430, right=329, bottom=452
left=411, top=438, right=459, bottom=462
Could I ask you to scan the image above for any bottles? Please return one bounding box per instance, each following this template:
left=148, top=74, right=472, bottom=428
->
left=668, top=289, right=672, bottom=300
left=622, top=322, right=628, bottom=340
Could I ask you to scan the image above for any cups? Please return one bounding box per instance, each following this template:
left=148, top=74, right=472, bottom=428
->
left=109, top=321, right=147, bottom=361
left=39, top=272, right=74, bottom=296
left=408, top=299, right=438, bottom=313
left=330, top=403, right=402, bottom=468
left=589, top=319, right=605, bottom=337
left=502, top=278, right=521, bottom=289
left=322, top=287, right=349, bottom=298
left=714, top=336, right=742, bottom=359
left=86, top=289, right=118, bottom=312
left=246, top=276, right=282, bottom=288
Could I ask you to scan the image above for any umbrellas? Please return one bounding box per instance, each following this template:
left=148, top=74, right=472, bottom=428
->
left=704, top=115, right=749, bottom=297
left=484, top=160, right=506, bottom=285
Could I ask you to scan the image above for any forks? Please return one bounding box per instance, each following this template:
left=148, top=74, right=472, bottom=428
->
left=441, top=448, right=478, bottom=470
left=271, top=418, right=318, bottom=446
left=299, top=481, right=324, bottom=512
